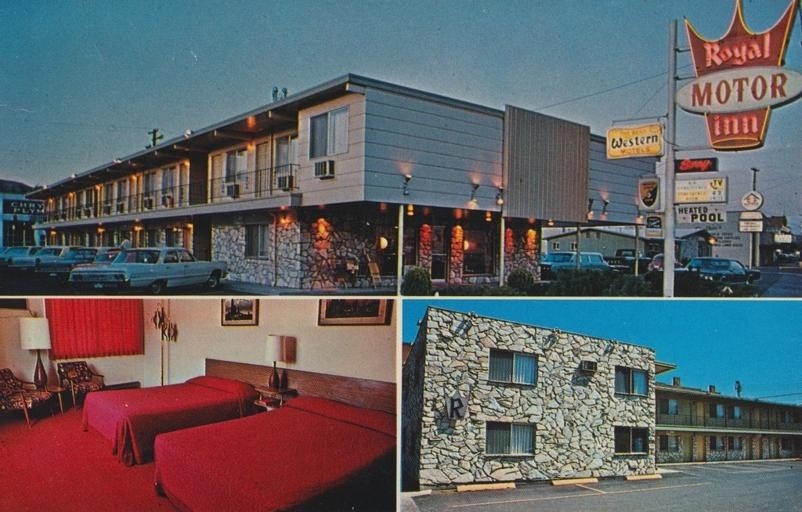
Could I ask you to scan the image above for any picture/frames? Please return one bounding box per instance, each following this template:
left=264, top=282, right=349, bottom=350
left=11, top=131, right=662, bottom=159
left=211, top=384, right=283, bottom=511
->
left=318, top=299, right=393, bottom=326
left=221, top=299, right=259, bottom=326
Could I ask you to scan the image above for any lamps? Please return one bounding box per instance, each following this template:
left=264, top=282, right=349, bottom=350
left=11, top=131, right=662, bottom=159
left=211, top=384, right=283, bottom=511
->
left=604, top=340, right=617, bottom=354
left=264, top=334, right=283, bottom=391
left=462, top=311, right=480, bottom=325
left=549, top=327, right=562, bottom=342
left=19, top=317, right=52, bottom=391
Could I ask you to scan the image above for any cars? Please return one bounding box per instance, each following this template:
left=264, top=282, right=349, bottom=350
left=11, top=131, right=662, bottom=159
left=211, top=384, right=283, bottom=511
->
left=649, top=253, right=685, bottom=273
left=674, top=257, right=761, bottom=286
left=0, top=246, right=156, bottom=272
left=541, top=251, right=613, bottom=274
left=67, top=247, right=227, bottom=295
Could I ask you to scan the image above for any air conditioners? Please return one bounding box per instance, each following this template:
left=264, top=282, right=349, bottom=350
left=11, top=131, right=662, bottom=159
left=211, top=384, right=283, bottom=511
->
left=314, top=160, right=336, bottom=179
left=74, top=193, right=173, bottom=219
left=276, top=177, right=293, bottom=191
left=580, top=361, right=598, bottom=373
left=226, top=184, right=239, bottom=199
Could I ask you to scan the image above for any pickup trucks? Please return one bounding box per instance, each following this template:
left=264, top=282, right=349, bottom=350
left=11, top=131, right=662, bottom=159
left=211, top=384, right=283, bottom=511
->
left=605, top=249, right=652, bottom=273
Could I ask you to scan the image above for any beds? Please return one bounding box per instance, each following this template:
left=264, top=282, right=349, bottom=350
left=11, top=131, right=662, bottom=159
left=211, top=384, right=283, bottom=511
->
left=80, top=375, right=260, bottom=467
left=153, top=396, right=396, bottom=512
left=38, top=384, right=66, bottom=415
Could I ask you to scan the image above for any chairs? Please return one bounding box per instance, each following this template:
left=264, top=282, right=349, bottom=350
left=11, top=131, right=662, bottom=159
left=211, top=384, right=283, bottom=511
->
left=0, top=368, right=56, bottom=430
left=57, top=361, right=104, bottom=408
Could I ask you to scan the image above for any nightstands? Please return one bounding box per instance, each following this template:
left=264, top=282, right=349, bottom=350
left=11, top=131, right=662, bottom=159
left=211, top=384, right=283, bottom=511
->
left=253, top=385, right=297, bottom=414
